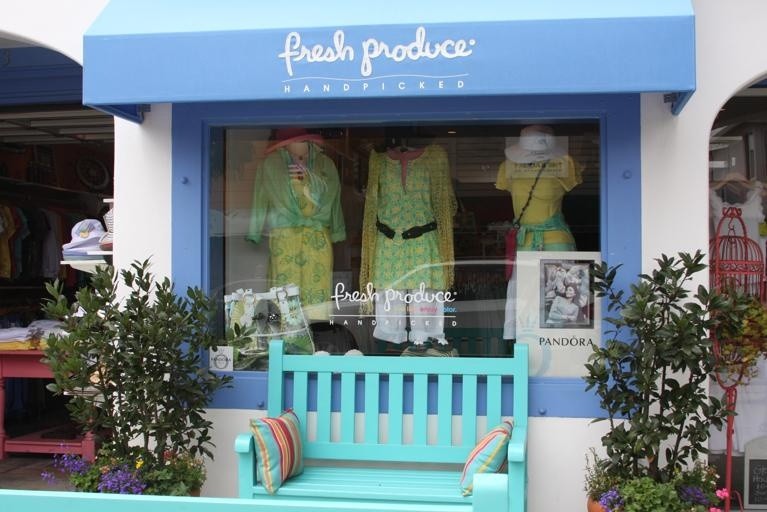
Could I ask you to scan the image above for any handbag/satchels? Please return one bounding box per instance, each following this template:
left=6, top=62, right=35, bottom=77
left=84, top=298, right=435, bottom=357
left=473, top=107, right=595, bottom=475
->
left=223, top=283, right=316, bottom=372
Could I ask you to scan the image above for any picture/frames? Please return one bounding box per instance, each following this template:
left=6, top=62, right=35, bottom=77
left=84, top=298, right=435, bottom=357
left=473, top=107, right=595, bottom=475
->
left=515, top=252, right=601, bottom=379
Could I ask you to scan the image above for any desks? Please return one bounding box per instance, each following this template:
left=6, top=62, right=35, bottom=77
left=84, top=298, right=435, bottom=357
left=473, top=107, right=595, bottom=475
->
left=2, top=322, right=100, bottom=464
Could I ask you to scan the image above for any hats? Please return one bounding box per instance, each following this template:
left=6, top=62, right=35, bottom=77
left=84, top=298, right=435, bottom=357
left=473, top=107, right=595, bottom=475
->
left=60, top=205, right=114, bottom=261
left=263, top=129, right=325, bottom=154
left=504, top=127, right=570, bottom=162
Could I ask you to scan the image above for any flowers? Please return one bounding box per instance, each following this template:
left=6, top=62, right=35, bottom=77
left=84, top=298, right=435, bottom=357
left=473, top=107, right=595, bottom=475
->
left=38, top=443, right=208, bottom=495
left=584, top=460, right=767, bottom=510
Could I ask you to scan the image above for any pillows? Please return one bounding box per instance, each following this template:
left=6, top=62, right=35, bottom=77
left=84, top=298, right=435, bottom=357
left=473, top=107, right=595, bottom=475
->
left=250, top=409, right=304, bottom=494
left=462, top=417, right=514, bottom=497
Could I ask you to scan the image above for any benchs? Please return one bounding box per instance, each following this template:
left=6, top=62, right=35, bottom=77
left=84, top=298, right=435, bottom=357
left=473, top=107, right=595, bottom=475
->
left=234, top=338, right=529, bottom=511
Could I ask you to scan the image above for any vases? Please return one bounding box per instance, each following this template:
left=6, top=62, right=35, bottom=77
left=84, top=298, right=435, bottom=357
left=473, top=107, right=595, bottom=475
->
left=587, top=498, right=615, bottom=512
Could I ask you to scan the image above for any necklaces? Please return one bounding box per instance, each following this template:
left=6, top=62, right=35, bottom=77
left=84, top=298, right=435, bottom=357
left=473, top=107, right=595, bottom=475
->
left=288, top=149, right=308, bottom=160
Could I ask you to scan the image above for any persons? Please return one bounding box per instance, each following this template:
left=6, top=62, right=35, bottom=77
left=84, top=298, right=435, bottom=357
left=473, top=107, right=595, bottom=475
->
left=245, top=128, right=345, bottom=305
left=545, top=263, right=565, bottom=295
left=496, top=126, right=582, bottom=340
left=547, top=284, right=579, bottom=324
left=359, top=142, right=458, bottom=347
